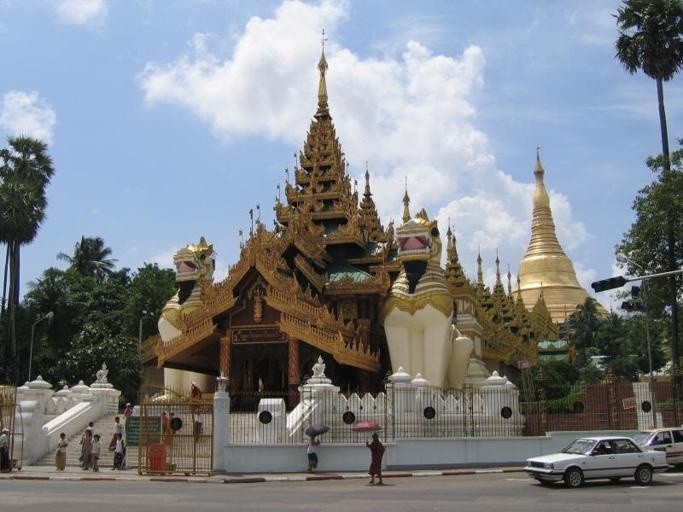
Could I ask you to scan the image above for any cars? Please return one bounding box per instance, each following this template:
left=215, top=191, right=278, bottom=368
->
left=524, top=437, right=668, bottom=487
left=635, top=427, right=682, bottom=469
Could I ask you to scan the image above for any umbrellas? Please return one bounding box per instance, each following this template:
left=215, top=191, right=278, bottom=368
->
left=352, top=421, right=383, bottom=442
left=305, top=426, right=329, bottom=440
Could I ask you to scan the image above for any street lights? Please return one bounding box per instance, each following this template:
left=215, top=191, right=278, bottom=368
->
left=27, top=311, right=53, bottom=380
left=138, top=310, right=154, bottom=386
left=616, top=252, right=658, bottom=427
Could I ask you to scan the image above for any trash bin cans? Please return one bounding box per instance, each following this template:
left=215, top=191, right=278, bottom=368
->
left=149, top=444, right=167, bottom=470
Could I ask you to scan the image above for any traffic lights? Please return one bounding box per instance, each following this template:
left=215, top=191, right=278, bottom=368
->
left=621, top=300, right=640, bottom=311
left=591, top=276, right=626, bottom=294
left=631, top=286, right=640, bottom=297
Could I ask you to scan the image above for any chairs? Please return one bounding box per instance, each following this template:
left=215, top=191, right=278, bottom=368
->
left=662, top=438, right=671, bottom=444
left=626, top=447, right=636, bottom=452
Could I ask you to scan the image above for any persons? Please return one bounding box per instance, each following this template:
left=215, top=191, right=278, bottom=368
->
left=191, top=383, right=202, bottom=414
left=56, top=433, right=68, bottom=469
left=169, top=412, right=176, bottom=440
left=162, top=412, right=168, bottom=440
left=366, top=434, right=384, bottom=484
left=307, top=437, right=319, bottom=472
left=0, top=428, right=14, bottom=470
left=124, top=403, right=132, bottom=433
left=80, top=417, right=127, bottom=471
